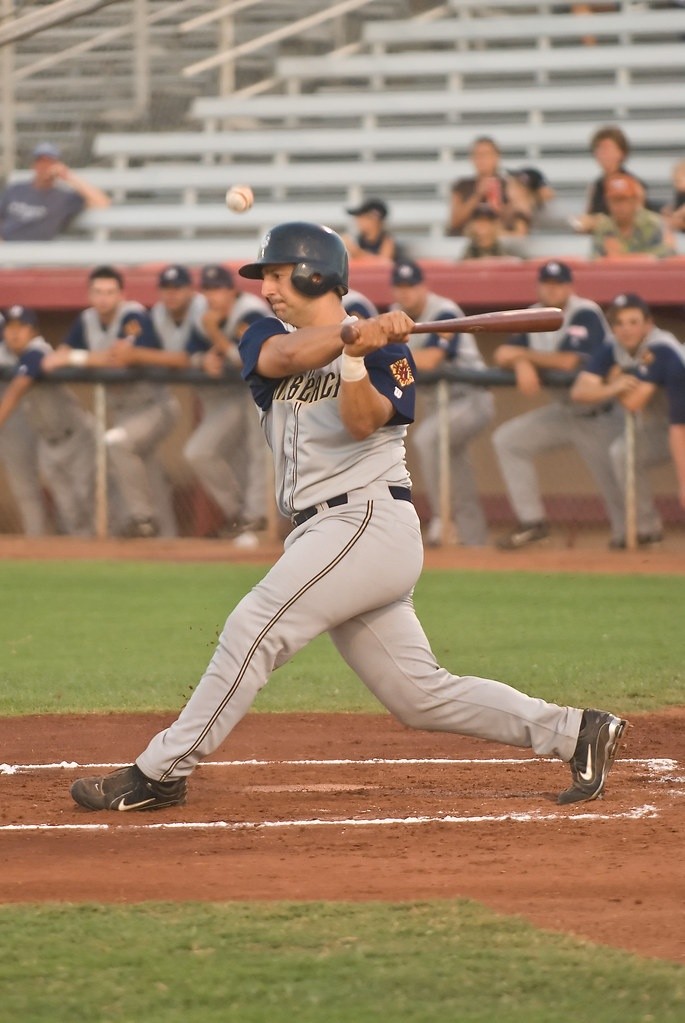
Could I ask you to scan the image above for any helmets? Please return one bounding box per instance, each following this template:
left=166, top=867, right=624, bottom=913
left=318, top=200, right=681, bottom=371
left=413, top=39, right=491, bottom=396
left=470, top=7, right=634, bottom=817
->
left=238, top=222, right=349, bottom=297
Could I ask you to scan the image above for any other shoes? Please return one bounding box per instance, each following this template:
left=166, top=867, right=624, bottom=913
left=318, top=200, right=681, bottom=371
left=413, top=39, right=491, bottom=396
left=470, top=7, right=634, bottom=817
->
left=496, top=522, right=549, bottom=549
left=610, top=532, right=667, bottom=553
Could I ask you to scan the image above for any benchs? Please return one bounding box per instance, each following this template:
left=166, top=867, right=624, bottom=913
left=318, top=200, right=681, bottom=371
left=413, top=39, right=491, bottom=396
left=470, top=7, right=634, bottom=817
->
left=0, top=0, right=685, bottom=257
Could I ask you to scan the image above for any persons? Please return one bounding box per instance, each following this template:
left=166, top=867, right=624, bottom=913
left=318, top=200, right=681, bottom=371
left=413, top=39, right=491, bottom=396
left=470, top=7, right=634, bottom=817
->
left=341, top=201, right=396, bottom=261
left=115, top=265, right=211, bottom=366
left=0, top=142, right=112, bottom=240
left=576, top=127, right=649, bottom=234
left=449, top=137, right=537, bottom=235
left=41, top=264, right=177, bottom=538
left=182, top=266, right=270, bottom=537
left=342, top=288, right=378, bottom=321
left=572, top=293, right=685, bottom=544
left=463, top=218, right=516, bottom=259
left=490, top=259, right=625, bottom=552
left=661, top=159, right=685, bottom=254
left=384, top=259, right=494, bottom=546
left=71, top=221, right=631, bottom=813
left=590, top=174, right=676, bottom=259
left=0, top=304, right=95, bottom=534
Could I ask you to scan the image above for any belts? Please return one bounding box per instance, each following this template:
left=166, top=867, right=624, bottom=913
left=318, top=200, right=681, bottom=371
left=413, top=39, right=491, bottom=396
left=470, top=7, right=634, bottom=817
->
left=290, top=486, right=412, bottom=527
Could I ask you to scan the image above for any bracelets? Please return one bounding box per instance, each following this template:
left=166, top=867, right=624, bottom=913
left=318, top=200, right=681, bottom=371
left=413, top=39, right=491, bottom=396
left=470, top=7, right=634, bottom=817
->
left=69, top=349, right=86, bottom=366
left=341, top=350, right=367, bottom=383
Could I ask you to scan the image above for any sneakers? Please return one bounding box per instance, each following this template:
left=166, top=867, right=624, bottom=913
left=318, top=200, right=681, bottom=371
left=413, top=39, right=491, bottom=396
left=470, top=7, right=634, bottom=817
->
left=71, top=764, right=188, bottom=811
left=557, top=707, right=629, bottom=806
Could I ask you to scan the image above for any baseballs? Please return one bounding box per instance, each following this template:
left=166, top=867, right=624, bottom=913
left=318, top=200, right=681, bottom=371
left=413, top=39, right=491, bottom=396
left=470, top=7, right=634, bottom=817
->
left=225, top=184, right=254, bottom=214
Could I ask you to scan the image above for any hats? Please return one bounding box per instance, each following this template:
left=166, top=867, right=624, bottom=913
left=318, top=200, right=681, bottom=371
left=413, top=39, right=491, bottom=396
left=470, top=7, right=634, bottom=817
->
left=200, top=264, right=231, bottom=290
left=605, top=175, right=645, bottom=197
left=8, top=305, right=39, bottom=325
left=392, top=261, right=421, bottom=286
left=609, top=293, right=646, bottom=309
left=509, top=169, right=542, bottom=188
left=159, top=265, right=188, bottom=287
left=33, top=143, right=61, bottom=159
left=347, top=199, right=387, bottom=219
left=539, top=260, right=573, bottom=282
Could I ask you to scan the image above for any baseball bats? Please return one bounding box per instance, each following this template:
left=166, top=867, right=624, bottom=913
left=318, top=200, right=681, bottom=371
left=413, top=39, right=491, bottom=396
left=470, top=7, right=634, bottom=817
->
left=341, top=307, right=568, bottom=340
